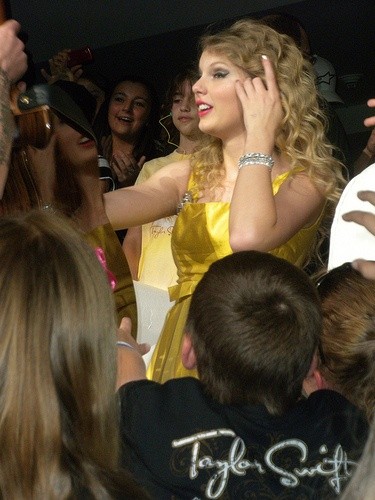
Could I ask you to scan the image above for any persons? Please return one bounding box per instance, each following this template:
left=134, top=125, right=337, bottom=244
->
left=0, top=1, right=374, bottom=500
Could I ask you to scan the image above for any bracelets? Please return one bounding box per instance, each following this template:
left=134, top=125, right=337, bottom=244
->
left=239, top=152, right=274, bottom=172
left=117, top=341, right=136, bottom=352
left=35, top=201, right=59, bottom=213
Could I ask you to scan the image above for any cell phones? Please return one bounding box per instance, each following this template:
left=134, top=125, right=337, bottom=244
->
left=65, top=47, right=94, bottom=70
